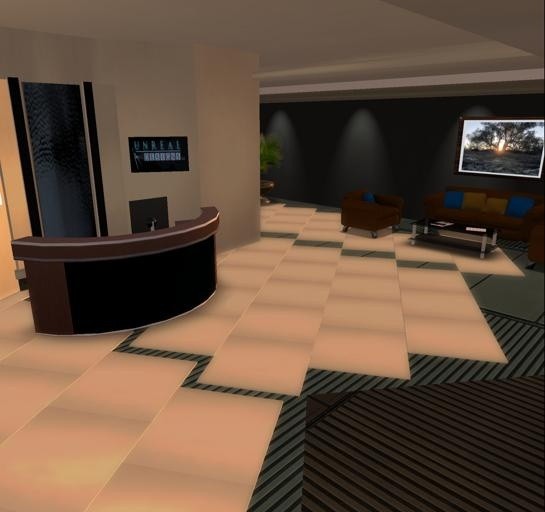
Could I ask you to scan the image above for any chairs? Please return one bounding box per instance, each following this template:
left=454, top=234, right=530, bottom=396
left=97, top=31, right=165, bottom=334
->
left=341, top=189, right=404, bottom=239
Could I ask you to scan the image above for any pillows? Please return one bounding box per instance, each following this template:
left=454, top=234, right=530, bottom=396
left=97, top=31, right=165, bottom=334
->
left=362, top=192, right=373, bottom=201
left=445, top=191, right=464, bottom=209
left=506, top=195, right=535, bottom=216
left=462, top=192, right=486, bottom=212
left=485, top=198, right=508, bottom=215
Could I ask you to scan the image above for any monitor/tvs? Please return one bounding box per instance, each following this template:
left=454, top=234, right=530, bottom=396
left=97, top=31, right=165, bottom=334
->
left=129, top=196, right=169, bottom=234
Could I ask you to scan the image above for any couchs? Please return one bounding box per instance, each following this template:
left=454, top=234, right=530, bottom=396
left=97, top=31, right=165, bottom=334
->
left=419, top=183, right=544, bottom=268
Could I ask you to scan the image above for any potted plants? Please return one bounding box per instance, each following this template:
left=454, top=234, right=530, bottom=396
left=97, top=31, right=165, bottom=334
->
left=260, top=132, right=283, bottom=206
